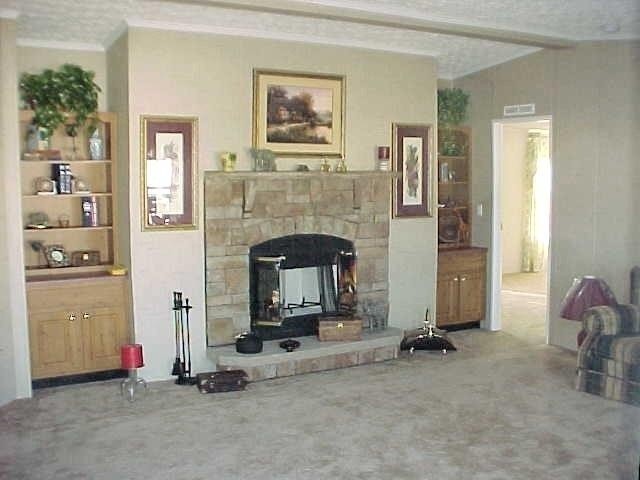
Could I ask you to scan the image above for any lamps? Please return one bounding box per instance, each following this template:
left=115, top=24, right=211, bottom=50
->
left=560, top=275, right=617, bottom=347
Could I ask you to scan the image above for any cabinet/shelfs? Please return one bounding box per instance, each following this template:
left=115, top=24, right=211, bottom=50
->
left=438, top=249, right=490, bottom=328
left=25, top=277, right=137, bottom=385
left=15, top=110, right=116, bottom=277
left=437, top=123, right=474, bottom=249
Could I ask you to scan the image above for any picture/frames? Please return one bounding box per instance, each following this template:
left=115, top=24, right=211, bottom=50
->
left=137, top=112, right=202, bottom=232
left=250, top=65, right=347, bottom=160
left=391, top=123, right=435, bottom=219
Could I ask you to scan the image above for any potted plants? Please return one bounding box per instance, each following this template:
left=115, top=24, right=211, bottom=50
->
left=12, top=54, right=108, bottom=161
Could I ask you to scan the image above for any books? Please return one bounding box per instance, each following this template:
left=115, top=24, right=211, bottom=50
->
left=440, top=161, right=467, bottom=184
left=51, top=164, right=66, bottom=194
left=81, top=197, right=97, bottom=226
left=64, top=164, right=71, bottom=193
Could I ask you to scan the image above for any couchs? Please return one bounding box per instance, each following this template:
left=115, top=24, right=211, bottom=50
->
left=576, top=265, right=640, bottom=408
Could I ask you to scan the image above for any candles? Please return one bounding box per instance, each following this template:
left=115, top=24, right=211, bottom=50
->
left=119, top=342, right=145, bottom=368
